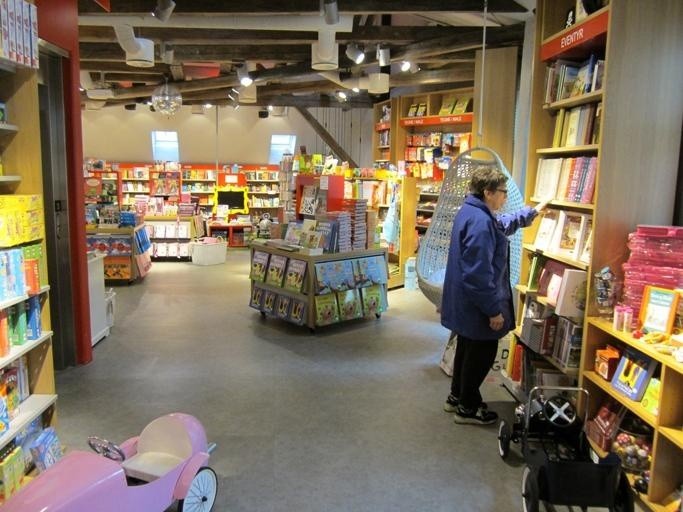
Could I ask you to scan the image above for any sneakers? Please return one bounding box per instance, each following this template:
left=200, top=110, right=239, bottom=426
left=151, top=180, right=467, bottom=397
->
left=444, top=395, right=487, bottom=412
left=454, top=405, right=498, bottom=424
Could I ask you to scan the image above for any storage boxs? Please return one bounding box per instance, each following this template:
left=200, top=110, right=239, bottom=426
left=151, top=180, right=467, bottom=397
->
left=190, top=242, right=228, bottom=266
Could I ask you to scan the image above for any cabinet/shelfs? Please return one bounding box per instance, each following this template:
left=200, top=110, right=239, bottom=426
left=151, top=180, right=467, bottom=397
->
left=0, top=58, right=59, bottom=507
left=102, top=162, right=280, bottom=208
left=583, top=316, right=683, bottom=512
left=506, top=0, right=683, bottom=417
left=373, top=98, right=397, bottom=171
left=399, top=87, right=475, bottom=257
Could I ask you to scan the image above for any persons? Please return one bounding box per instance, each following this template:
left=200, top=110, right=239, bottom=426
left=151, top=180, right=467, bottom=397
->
left=438, top=165, right=557, bottom=431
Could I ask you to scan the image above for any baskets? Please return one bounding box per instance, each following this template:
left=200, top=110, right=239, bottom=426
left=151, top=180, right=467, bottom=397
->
left=190, top=235, right=227, bottom=265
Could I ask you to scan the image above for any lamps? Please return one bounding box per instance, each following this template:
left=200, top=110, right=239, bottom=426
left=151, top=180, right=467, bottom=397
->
left=115, top=0, right=411, bottom=116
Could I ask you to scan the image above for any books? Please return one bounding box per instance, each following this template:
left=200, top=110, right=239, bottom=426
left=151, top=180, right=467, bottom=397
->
left=611, top=343, right=659, bottom=401
left=661, top=480, right=683, bottom=512
left=182, top=165, right=283, bottom=245
left=593, top=395, right=629, bottom=437
left=0, top=0, right=40, bottom=72
left=83, top=159, right=191, bottom=278
left=0, top=193, right=64, bottom=504
left=250, top=176, right=370, bottom=326
left=502, top=2, right=610, bottom=416
left=372, top=90, right=474, bottom=315
left=639, top=284, right=679, bottom=339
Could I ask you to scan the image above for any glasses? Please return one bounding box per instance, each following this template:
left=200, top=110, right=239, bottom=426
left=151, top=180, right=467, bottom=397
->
left=497, top=189, right=507, bottom=193
